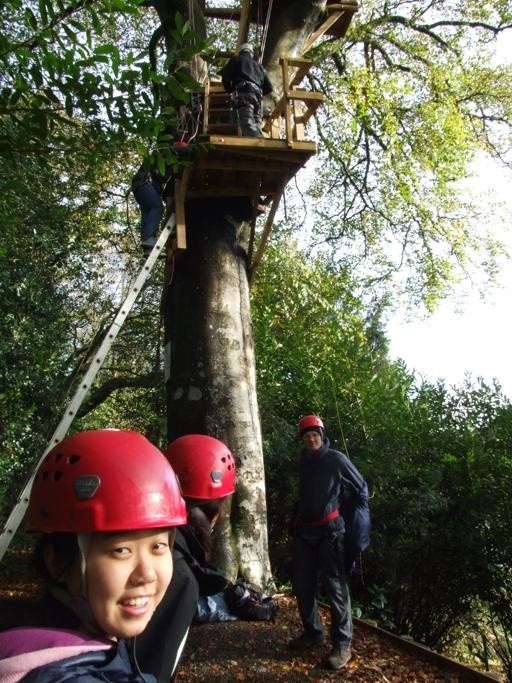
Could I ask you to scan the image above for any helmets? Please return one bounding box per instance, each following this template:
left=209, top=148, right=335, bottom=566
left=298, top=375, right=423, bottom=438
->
left=296, top=413, right=325, bottom=432
left=237, top=42, right=257, bottom=54
left=163, top=430, right=240, bottom=500
left=23, top=426, right=191, bottom=533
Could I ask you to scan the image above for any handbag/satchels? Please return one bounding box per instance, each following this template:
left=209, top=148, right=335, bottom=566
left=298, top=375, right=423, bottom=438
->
left=227, top=581, right=277, bottom=622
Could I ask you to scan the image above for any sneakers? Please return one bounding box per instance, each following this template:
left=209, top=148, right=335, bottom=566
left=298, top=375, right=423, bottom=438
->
left=287, top=629, right=325, bottom=651
left=140, top=235, right=159, bottom=248
left=142, top=248, right=169, bottom=258
left=322, top=639, right=354, bottom=670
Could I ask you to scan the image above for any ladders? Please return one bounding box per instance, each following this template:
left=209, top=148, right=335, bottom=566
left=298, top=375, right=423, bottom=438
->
left=0, top=212, right=177, bottom=562
left=202, top=76, right=245, bottom=137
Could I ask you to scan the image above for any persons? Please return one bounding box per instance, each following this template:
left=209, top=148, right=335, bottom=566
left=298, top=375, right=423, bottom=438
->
left=127, top=432, right=237, bottom=682
left=288, top=414, right=373, bottom=670
left=0, top=426, right=191, bottom=682
